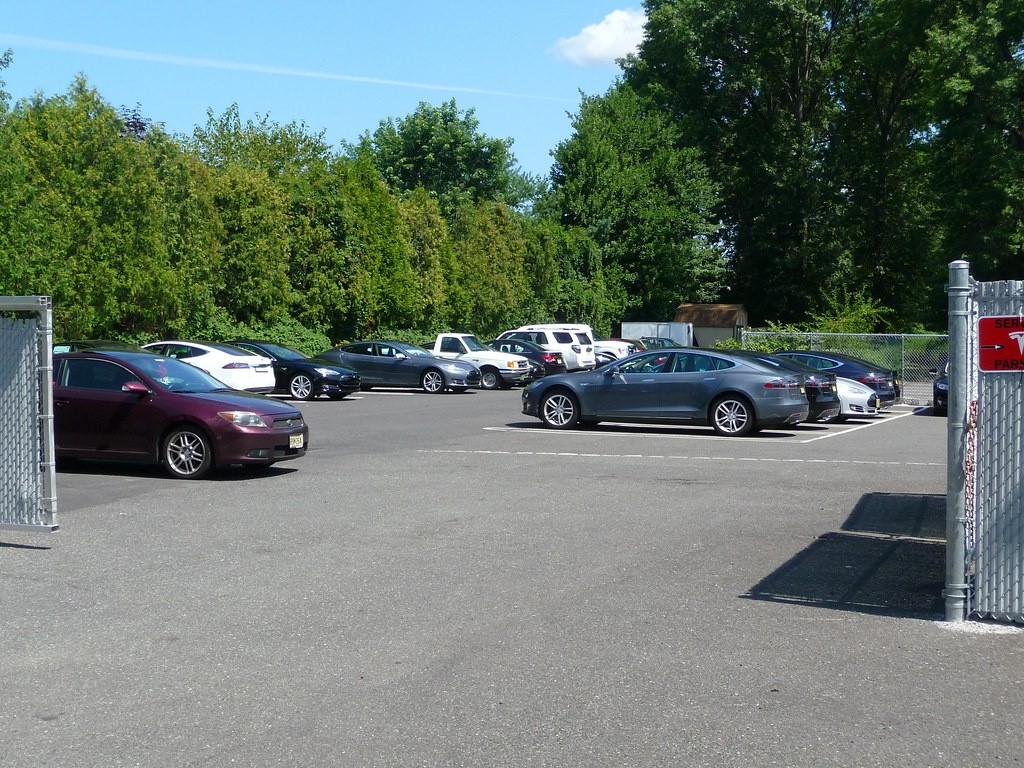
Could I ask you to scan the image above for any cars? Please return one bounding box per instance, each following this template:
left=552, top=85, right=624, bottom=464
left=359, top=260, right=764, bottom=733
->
left=52, top=340, right=309, bottom=480
left=222, top=341, right=361, bottom=401
left=140, top=341, right=276, bottom=395
left=928, top=360, right=948, bottom=414
left=314, top=341, right=481, bottom=394
left=483, top=339, right=567, bottom=384
left=770, top=350, right=901, bottom=420
left=522, top=349, right=810, bottom=437
left=646, top=349, right=841, bottom=423
left=595, top=337, right=683, bottom=370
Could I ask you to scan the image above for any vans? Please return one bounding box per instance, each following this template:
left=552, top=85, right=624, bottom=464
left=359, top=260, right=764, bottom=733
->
left=496, top=324, right=595, bottom=372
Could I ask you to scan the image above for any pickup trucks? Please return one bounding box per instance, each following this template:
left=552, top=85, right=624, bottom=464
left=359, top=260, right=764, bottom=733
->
left=366, top=333, right=531, bottom=390
